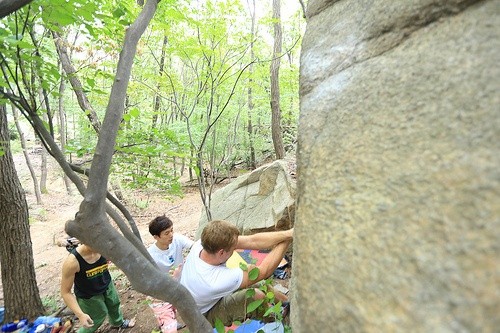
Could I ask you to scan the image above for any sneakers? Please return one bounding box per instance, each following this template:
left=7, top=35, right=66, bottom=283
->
left=110, top=320, right=135, bottom=328
